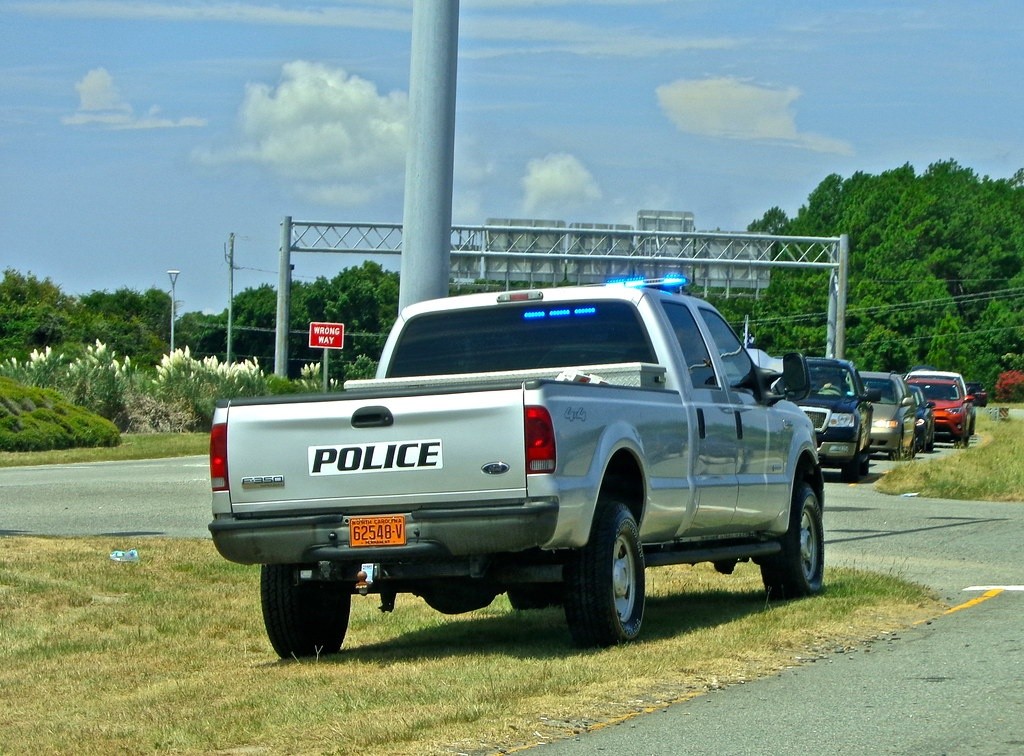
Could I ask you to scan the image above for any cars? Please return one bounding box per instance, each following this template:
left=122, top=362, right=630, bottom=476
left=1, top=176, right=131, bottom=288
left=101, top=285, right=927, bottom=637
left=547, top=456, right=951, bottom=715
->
left=908, top=384, right=936, bottom=453
left=902, top=369, right=987, bottom=447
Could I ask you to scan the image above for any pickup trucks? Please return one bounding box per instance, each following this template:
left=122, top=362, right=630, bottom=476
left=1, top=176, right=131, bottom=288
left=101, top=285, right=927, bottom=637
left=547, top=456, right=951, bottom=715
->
left=204, top=271, right=830, bottom=665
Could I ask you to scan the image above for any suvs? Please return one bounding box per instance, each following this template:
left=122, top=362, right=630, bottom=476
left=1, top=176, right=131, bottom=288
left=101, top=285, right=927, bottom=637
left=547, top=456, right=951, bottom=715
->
left=766, top=353, right=881, bottom=484
left=856, top=364, right=914, bottom=462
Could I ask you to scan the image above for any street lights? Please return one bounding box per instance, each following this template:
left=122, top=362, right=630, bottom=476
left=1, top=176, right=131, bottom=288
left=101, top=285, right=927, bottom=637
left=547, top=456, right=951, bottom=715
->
left=166, top=270, right=182, bottom=355
